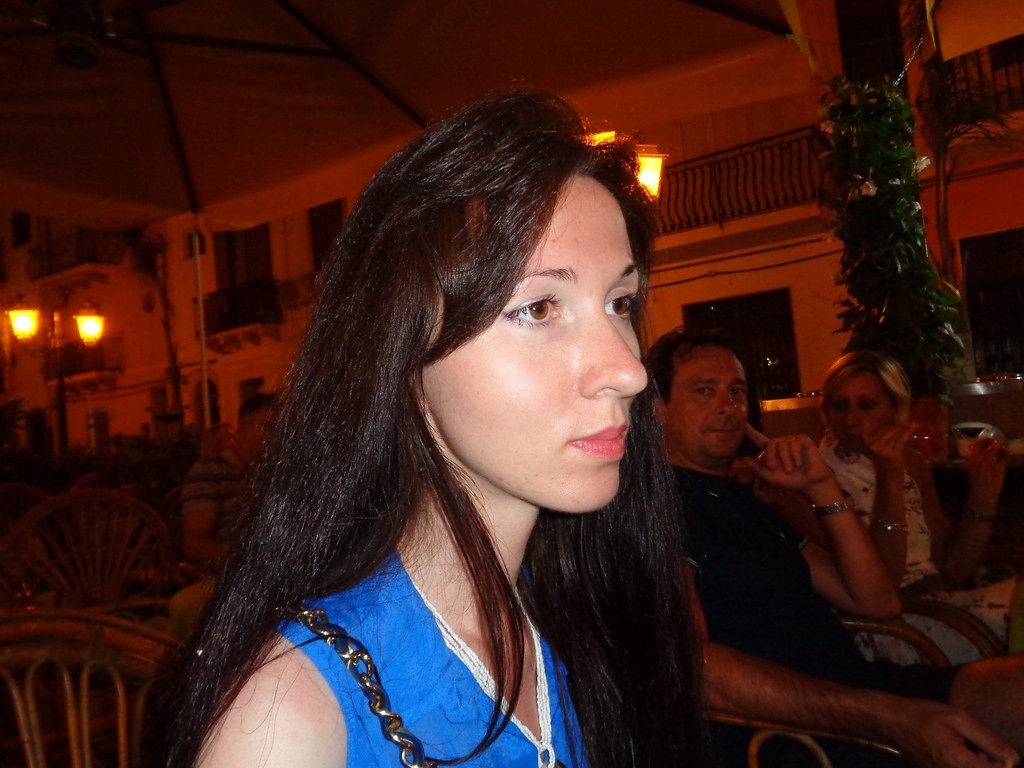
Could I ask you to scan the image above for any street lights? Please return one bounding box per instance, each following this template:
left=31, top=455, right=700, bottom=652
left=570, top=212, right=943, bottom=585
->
left=6, top=283, right=107, bottom=481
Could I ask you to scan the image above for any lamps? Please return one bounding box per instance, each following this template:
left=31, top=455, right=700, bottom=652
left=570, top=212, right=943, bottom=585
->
left=76, top=289, right=105, bottom=349
left=6, top=292, right=39, bottom=344
left=622, top=144, right=670, bottom=201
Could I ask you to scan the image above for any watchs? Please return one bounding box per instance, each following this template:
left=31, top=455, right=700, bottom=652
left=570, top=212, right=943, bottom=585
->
left=810, top=494, right=855, bottom=516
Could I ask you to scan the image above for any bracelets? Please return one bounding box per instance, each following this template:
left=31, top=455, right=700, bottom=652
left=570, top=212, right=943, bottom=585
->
left=871, top=516, right=909, bottom=536
left=967, top=510, right=995, bottom=522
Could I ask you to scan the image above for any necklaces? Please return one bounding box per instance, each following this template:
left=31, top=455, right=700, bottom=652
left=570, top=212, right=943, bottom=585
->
left=416, top=585, right=555, bottom=768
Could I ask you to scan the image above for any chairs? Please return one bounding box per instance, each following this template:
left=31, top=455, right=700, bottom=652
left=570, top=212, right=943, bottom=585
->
left=0, top=472, right=1006, bottom=768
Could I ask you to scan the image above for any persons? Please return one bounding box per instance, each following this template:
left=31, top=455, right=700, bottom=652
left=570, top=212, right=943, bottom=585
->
left=647, top=327, right=1023, bottom=767
left=141, top=84, right=710, bottom=768
left=786, top=349, right=1024, bottom=666
